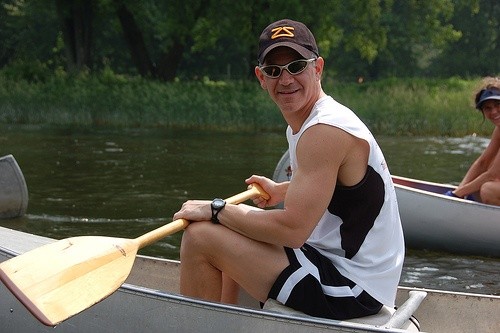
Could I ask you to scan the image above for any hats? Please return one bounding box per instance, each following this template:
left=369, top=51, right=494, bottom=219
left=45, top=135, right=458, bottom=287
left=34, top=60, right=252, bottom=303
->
left=475, top=88, right=500, bottom=108
left=257, top=19, right=319, bottom=66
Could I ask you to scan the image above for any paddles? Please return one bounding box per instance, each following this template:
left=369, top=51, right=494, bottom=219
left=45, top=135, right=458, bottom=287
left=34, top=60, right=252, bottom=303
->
left=0, top=180, right=272, bottom=328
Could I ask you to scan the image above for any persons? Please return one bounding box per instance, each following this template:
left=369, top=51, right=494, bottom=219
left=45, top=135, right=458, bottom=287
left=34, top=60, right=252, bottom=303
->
left=172, top=19, right=405, bottom=321
left=446, top=76, right=500, bottom=207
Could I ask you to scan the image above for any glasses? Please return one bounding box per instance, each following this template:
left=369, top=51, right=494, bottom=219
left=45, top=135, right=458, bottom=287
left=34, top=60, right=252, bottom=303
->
left=259, top=58, right=316, bottom=79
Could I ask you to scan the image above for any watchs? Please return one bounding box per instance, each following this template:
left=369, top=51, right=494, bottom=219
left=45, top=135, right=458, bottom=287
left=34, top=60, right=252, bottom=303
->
left=210, top=198, right=226, bottom=224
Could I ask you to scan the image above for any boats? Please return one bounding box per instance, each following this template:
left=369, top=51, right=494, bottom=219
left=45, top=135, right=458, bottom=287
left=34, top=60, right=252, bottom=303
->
left=273, top=149, right=500, bottom=256
left=0, top=154, right=500, bottom=333
